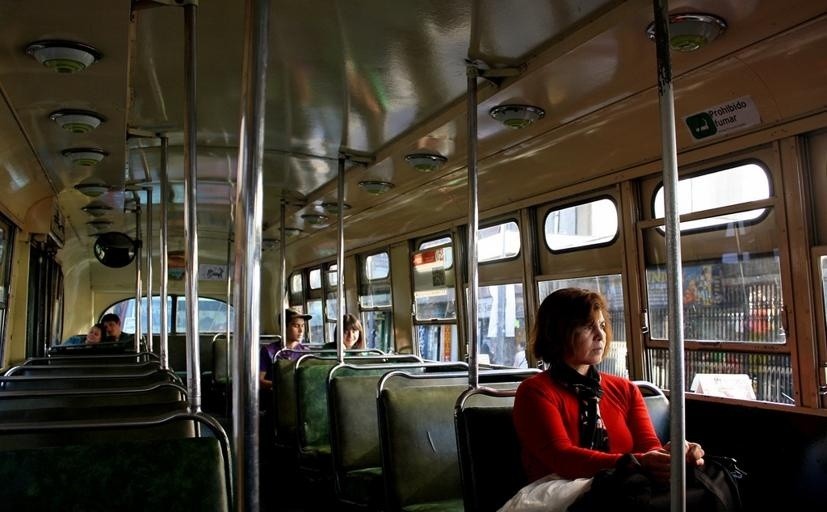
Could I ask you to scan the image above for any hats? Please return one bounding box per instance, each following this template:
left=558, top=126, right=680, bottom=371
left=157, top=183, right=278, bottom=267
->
left=278, top=307, right=313, bottom=327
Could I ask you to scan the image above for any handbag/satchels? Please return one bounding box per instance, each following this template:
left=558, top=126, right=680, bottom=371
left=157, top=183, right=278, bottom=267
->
left=566, top=452, right=747, bottom=512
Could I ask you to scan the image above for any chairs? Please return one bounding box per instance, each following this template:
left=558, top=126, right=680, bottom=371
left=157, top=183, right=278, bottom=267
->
left=211, top=332, right=282, bottom=418
left=146, top=334, right=216, bottom=385
left=0, top=409, right=235, bottom=511
left=326, top=361, right=468, bottom=512
left=0, top=382, right=188, bottom=438
left=20, top=351, right=160, bottom=372
left=455, top=380, right=669, bottom=512
left=48, top=338, right=146, bottom=366
left=1, top=367, right=184, bottom=397
left=274, top=348, right=385, bottom=491
left=294, top=353, right=424, bottom=501
left=376, top=368, right=542, bottom=512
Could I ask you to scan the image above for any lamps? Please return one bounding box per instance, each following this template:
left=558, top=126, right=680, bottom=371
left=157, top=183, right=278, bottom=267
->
left=278, top=228, right=301, bottom=238
left=23, top=38, right=105, bottom=73
left=263, top=238, right=280, bottom=250
left=301, top=214, right=329, bottom=225
left=489, top=104, right=546, bottom=131
left=322, top=203, right=351, bottom=214
left=61, top=148, right=109, bottom=166
left=86, top=221, right=113, bottom=230
left=404, top=154, right=448, bottom=173
left=81, top=206, right=113, bottom=217
left=357, top=181, right=396, bottom=195
left=645, top=12, right=728, bottom=53
left=74, top=184, right=112, bottom=197
left=48, top=109, right=107, bottom=135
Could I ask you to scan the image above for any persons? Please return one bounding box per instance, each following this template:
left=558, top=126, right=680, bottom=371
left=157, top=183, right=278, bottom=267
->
left=514, top=287, right=705, bottom=512
left=260, top=308, right=313, bottom=387
left=63, top=322, right=104, bottom=345
left=102, top=313, right=134, bottom=342
left=321, top=314, right=365, bottom=357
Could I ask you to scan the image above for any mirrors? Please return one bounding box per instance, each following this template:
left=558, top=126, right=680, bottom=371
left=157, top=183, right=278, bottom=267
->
left=94, top=232, right=137, bottom=268
